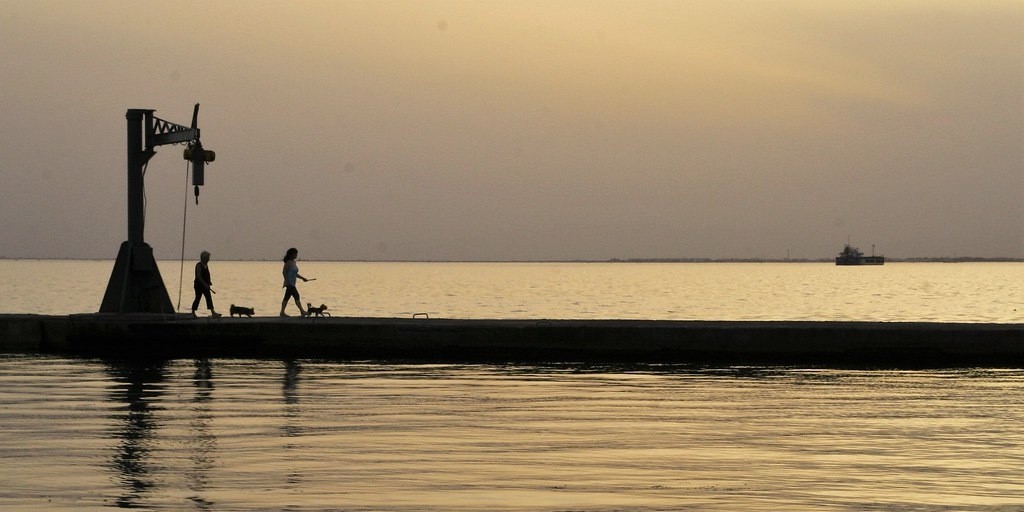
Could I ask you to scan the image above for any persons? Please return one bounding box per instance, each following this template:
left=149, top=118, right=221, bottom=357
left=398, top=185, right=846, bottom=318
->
left=280, top=248, right=308, bottom=316
left=191, top=251, right=222, bottom=317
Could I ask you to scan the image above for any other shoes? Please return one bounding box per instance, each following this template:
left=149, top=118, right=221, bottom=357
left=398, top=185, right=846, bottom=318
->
left=212, top=313, right=221, bottom=317
left=279, top=312, right=288, bottom=316
left=192, top=313, right=196, bottom=316
left=301, top=312, right=309, bottom=316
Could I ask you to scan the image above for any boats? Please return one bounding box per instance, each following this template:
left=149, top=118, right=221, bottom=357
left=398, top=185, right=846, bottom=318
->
left=836, top=245, right=885, bottom=266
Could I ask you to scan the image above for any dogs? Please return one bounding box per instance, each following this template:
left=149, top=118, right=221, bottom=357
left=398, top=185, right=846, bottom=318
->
left=306, top=303, right=328, bottom=318
left=230, top=304, right=255, bottom=318
